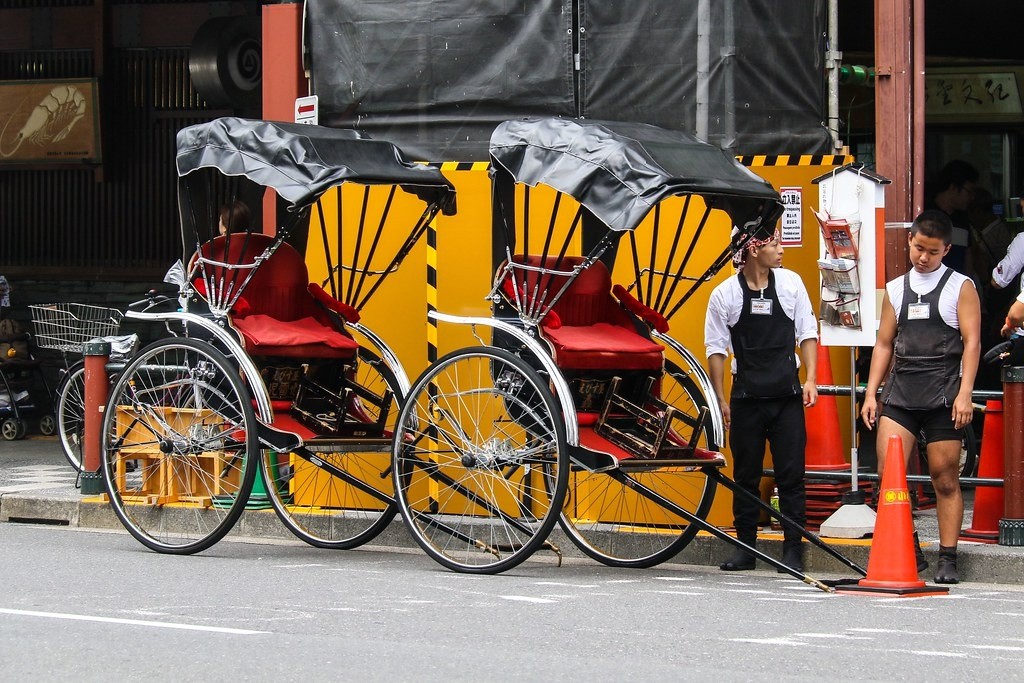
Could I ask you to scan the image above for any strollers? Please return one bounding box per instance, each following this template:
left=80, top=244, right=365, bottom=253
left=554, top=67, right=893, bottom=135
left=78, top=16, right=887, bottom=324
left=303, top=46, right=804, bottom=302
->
left=0, top=303, right=63, bottom=441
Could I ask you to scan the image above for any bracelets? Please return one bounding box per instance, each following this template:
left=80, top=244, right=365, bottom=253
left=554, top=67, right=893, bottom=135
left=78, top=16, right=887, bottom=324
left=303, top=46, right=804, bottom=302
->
left=1006, top=317, right=1016, bottom=329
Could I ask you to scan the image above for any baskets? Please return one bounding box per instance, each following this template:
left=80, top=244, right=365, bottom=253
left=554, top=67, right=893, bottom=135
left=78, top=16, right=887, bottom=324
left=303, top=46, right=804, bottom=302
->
left=28, top=303, right=124, bottom=352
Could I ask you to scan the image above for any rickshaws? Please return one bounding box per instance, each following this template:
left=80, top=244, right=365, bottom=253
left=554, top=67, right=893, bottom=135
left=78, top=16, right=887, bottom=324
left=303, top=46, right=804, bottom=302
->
left=99, top=117, right=562, bottom=571
left=391, top=118, right=869, bottom=594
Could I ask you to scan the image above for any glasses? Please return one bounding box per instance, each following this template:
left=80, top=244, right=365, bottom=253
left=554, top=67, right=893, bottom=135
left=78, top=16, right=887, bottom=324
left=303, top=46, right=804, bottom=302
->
left=961, top=185, right=978, bottom=198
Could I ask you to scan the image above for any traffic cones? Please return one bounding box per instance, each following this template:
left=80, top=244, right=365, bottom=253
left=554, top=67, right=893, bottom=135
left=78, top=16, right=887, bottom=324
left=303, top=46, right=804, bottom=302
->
left=834, top=433, right=951, bottom=598
left=797, top=331, right=875, bottom=532
left=956, top=398, right=1005, bottom=544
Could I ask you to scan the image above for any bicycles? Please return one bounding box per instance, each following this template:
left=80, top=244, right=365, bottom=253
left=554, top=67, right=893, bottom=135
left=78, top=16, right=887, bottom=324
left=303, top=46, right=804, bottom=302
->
left=28, top=300, right=218, bottom=489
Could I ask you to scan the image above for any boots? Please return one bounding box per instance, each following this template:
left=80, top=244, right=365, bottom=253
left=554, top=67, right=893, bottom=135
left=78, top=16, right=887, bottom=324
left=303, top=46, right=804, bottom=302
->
left=777, top=539, right=803, bottom=573
left=911, top=531, right=929, bottom=572
left=718, top=534, right=758, bottom=570
left=933, top=544, right=960, bottom=584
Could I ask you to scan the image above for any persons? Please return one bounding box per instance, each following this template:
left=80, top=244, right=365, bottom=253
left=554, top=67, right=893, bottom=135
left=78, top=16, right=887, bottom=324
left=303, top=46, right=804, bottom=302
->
left=861, top=209, right=980, bottom=585
left=922, top=166, right=1024, bottom=365
left=219, top=201, right=250, bottom=235
left=704, top=225, right=819, bottom=574
